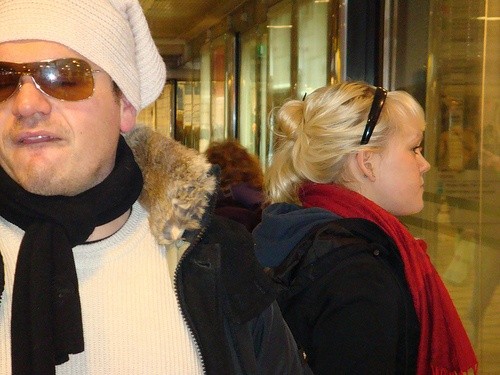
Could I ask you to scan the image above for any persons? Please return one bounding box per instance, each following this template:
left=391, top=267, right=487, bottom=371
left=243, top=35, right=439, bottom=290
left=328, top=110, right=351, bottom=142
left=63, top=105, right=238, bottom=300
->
left=256, top=81, right=476, bottom=374
left=201, top=136, right=265, bottom=224
left=0, top=0, right=313, bottom=375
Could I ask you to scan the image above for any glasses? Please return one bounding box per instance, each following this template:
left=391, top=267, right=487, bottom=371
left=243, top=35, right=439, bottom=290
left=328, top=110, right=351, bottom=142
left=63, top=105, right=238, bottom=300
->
left=0, top=58, right=103, bottom=102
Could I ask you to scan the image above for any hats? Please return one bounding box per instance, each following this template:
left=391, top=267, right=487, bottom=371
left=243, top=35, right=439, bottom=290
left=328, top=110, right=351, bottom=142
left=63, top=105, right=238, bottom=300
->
left=0, top=0, right=166, bottom=112
